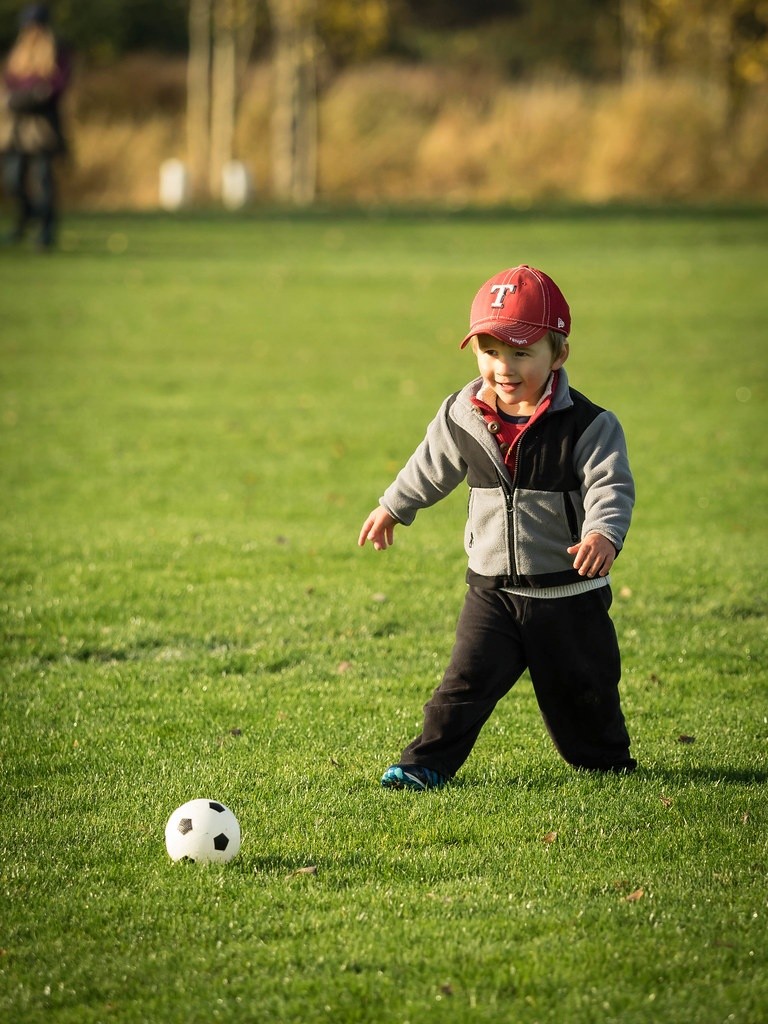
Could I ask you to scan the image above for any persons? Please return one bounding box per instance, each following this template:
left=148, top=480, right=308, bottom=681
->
left=357, top=265, right=634, bottom=791
left=0, top=0, right=90, bottom=254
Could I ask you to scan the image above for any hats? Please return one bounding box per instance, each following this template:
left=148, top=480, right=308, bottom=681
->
left=460, top=265, right=572, bottom=345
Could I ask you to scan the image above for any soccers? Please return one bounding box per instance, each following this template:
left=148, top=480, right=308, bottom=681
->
left=164, top=797, right=241, bottom=864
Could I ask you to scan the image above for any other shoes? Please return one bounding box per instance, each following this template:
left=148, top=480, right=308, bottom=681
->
left=382, top=762, right=447, bottom=793
